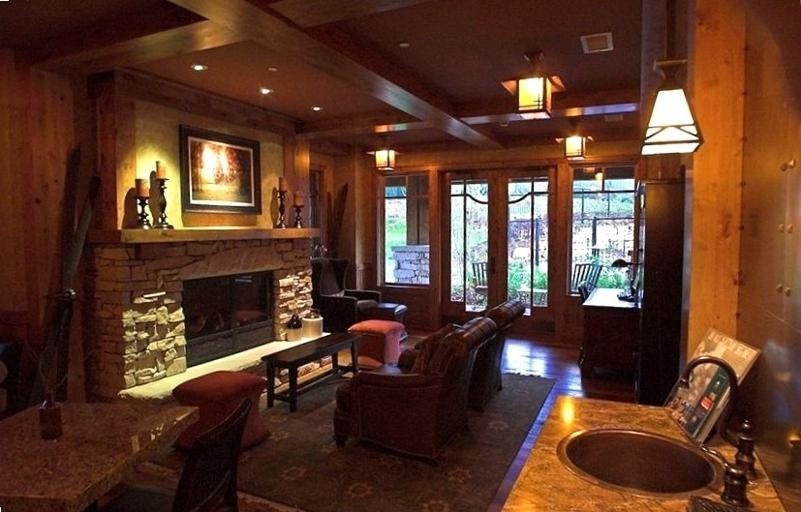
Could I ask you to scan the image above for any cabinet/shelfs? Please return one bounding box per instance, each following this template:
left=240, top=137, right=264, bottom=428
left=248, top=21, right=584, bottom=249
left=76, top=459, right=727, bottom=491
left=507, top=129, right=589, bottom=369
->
left=633, top=179, right=684, bottom=402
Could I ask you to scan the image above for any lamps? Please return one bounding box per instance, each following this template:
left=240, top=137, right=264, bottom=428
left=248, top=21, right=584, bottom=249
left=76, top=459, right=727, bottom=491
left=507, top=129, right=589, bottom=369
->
left=497, top=49, right=569, bottom=120
left=365, top=135, right=404, bottom=171
left=637, top=57, right=704, bottom=157
left=554, top=117, right=596, bottom=161
left=611, top=259, right=638, bottom=302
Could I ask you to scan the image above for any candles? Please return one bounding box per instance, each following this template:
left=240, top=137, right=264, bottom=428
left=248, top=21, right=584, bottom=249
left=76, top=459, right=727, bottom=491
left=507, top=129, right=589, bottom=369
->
left=279, top=177, right=288, bottom=191
left=293, top=189, right=305, bottom=206
left=155, top=159, right=168, bottom=179
left=135, top=178, right=149, bottom=196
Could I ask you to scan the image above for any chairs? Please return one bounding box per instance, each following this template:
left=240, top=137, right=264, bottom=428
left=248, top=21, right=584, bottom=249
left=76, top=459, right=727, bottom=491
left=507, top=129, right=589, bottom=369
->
left=98, top=397, right=253, bottom=512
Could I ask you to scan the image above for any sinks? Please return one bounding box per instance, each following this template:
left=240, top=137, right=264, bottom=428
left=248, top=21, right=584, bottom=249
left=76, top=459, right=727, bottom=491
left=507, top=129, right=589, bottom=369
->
left=557, top=427, right=725, bottom=499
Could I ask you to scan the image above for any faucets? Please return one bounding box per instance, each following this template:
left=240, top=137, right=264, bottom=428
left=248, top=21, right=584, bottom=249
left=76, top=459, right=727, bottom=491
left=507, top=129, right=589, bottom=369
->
left=680, top=355, right=757, bottom=481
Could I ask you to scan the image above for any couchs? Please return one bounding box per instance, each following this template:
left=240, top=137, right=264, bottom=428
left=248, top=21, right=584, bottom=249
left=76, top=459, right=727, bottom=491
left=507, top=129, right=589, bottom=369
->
left=312, top=257, right=380, bottom=327
left=397, top=299, right=527, bottom=415
left=331, top=316, right=499, bottom=461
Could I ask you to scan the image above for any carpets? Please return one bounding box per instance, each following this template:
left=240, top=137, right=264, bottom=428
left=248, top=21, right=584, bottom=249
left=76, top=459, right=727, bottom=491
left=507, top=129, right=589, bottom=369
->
left=147, top=367, right=557, bottom=512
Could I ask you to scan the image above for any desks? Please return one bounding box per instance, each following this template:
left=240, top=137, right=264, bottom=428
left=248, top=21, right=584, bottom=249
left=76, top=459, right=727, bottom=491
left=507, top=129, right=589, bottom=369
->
left=581, top=288, right=641, bottom=383
left=0, top=401, right=202, bottom=512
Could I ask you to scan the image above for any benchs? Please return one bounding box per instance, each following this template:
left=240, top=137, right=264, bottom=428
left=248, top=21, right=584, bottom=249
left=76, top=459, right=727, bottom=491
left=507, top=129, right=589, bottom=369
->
left=261, top=333, right=361, bottom=412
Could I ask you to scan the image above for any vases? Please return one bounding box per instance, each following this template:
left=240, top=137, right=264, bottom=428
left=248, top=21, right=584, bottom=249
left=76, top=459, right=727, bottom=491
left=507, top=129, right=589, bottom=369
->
left=38, top=391, right=63, bottom=440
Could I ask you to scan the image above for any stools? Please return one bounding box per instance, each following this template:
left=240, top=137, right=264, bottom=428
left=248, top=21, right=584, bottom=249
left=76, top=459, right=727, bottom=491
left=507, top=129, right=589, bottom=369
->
left=172, top=370, right=272, bottom=454
left=349, top=319, right=406, bottom=365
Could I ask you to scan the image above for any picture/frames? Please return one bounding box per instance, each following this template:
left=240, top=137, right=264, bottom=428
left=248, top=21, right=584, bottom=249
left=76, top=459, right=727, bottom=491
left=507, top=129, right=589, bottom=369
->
left=178, top=123, right=262, bottom=216
